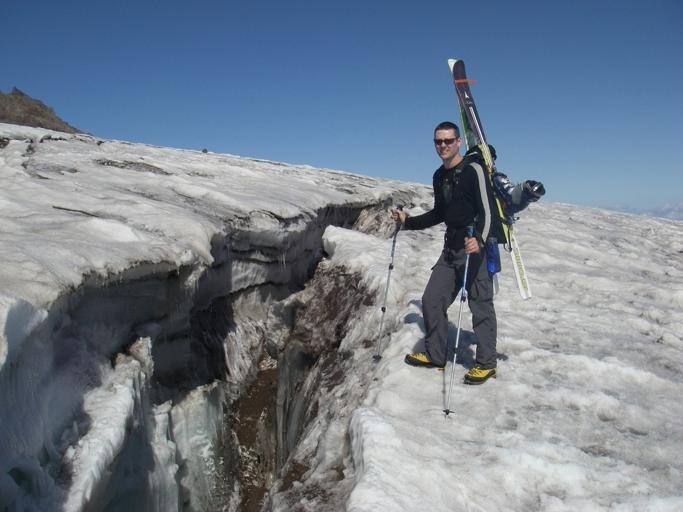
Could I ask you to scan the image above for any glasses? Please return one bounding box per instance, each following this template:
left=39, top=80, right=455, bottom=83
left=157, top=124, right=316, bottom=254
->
left=434, top=138, right=456, bottom=144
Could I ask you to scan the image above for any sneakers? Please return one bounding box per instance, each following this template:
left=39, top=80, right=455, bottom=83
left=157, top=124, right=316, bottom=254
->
left=406, top=352, right=444, bottom=369
left=464, top=365, right=496, bottom=384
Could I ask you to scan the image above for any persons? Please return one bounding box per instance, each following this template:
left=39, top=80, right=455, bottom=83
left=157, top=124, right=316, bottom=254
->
left=391, top=121, right=501, bottom=384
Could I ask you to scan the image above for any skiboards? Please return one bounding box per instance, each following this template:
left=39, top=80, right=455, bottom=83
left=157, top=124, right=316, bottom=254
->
left=447, top=59, right=532, bottom=299
